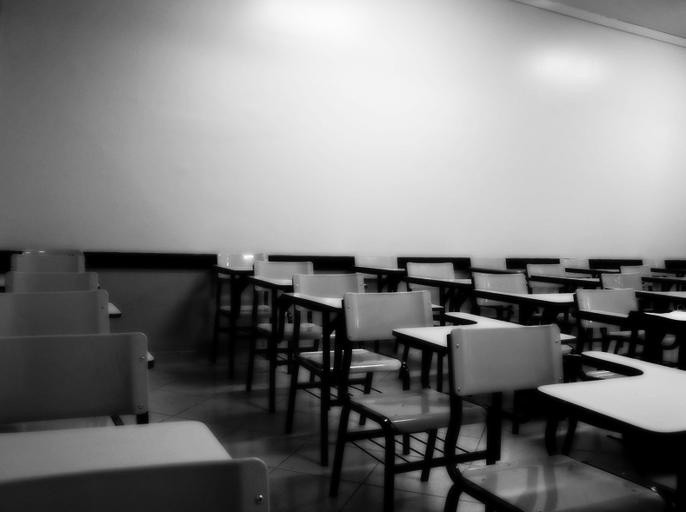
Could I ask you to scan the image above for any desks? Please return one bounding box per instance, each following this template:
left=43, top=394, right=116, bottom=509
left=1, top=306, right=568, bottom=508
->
left=249, top=274, right=368, bottom=415
left=537, top=351, right=686, bottom=512
left=605, top=287, right=686, bottom=310
left=407, top=275, right=471, bottom=312
left=0, top=420, right=232, bottom=483
left=108, top=302, right=122, bottom=319
left=473, top=288, right=576, bottom=325
left=578, top=310, right=686, bottom=369
left=111, top=351, right=155, bottom=425
left=392, top=312, right=577, bottom=461
left=641, top=276, right=686, bottom=291
left=215, top=265, right=253, bottom=383
left=355, top=266, right=406, bottom=292
left=275, top=293, right=444, bottom=469
left=531, top=274, right=600, bottom=289
left=471, top=267, right=526, bottom=274
left=565, top=267, right=620, bottom=277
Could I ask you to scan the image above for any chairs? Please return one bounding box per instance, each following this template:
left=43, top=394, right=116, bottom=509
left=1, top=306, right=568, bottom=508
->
left=560, top=254, right=590, bottom=270
left=0, top=457, right=270, bottom=512
left=470, top=253, right=506, bottom=277
left=23, top=248, right=81, bottom=255
left=619, top=264, right=650, bottom=274
left=11, top=254, right=85, bottom=272
left=245, top=262, right=331, bottom=410
left=329, top=290, right=485, bottom=511
left=642, top=255, right=665, bottom=270
left=284, top=274, right=411, bottom=455
left=353, top=253, right=399, bottom=293
left=5, top=272, right=98, bottom=293
left=209, top=246, right=277, bottom=368
left=525, top=263, right=564, bottom=293
left=469, top=268, right=533, bottom=323
left=404, top=261, right=455, bottom=326
left=0, top=289, right=110, bottom=337
left=444, top=324, right=663, bottom=512
left=0, top=332, right=149, bottom=424
left=573, top=288, right=638, bottom=355
left=599, top=272, right=643, bottom=292
